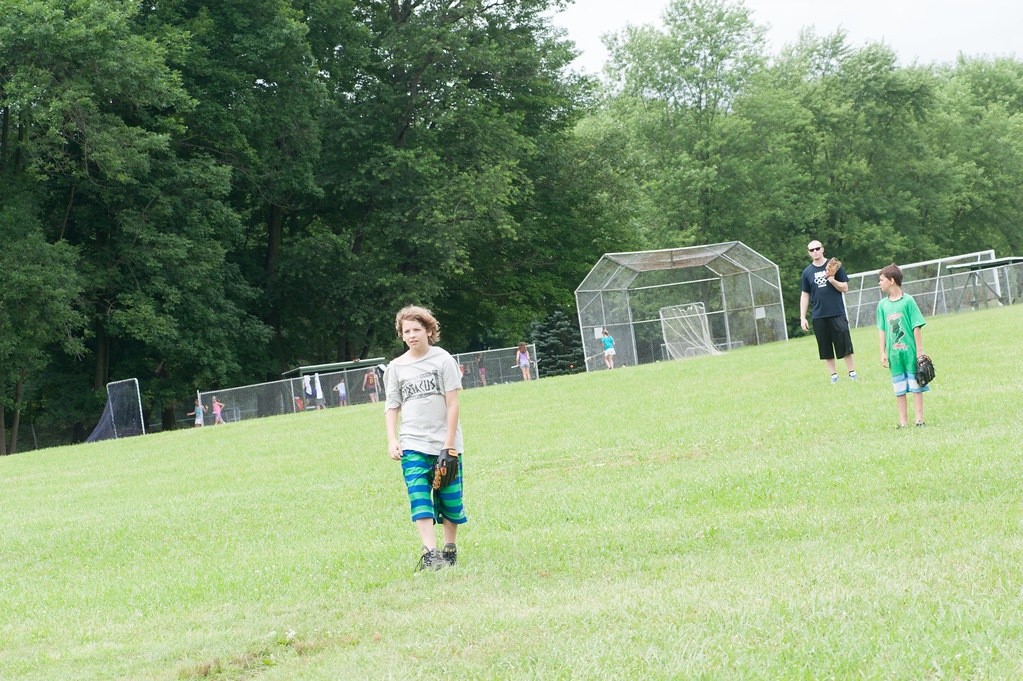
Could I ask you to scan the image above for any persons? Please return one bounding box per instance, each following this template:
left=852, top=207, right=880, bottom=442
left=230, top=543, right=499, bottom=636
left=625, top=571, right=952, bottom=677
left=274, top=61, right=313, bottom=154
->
left=188, top=342, right=533, bottom=427
left=601, top=330, right=616, bottom=370
left=800, top=240, right=859, bottom=384
left=383, top=306, right=467, bottom=574
left=877, top=264, right=935, bottom=430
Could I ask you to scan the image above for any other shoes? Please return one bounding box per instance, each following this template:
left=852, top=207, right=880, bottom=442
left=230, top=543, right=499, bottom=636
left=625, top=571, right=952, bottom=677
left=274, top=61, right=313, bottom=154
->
left=831, top=373, right=840, bottom=385
left=848, top=370, right=858, bottom=380
left=418, top=546, right=457, bottom=571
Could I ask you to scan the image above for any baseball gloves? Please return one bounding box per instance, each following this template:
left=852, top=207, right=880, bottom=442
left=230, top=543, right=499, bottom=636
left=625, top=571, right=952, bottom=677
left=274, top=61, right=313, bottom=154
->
left=915, top=355, right=935, bottom=388
left=432, top=448, right=459, bottom=490
left=824, top=257, right=842, bottom=279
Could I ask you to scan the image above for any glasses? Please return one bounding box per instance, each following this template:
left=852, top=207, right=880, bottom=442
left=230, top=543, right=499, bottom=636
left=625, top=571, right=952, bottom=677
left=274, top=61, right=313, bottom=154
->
left=809, top=247, right=823, bottom=252
